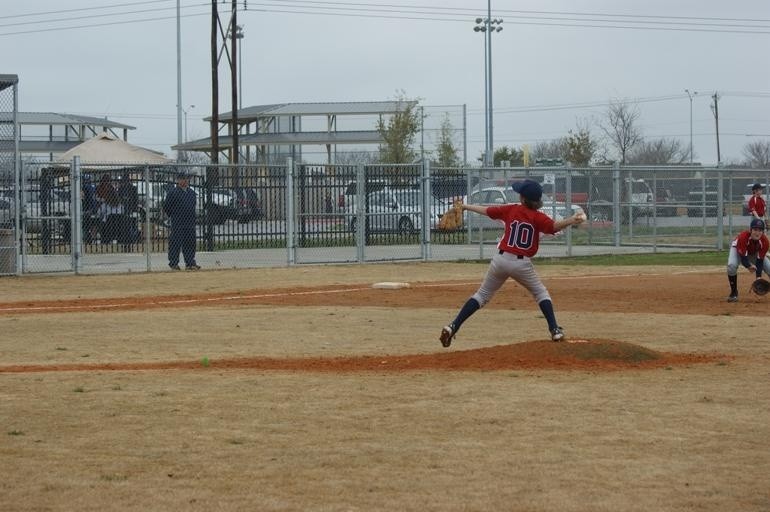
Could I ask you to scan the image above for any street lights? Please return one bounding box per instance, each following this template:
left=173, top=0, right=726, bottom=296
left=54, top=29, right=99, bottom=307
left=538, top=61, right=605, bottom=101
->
left=222, top=19, right=249, bottom=164
left=474, top=14, right=505, bottom=154
left=174, top=101, right=196, bottom=165
left=684, top=86, right=699, bottom=194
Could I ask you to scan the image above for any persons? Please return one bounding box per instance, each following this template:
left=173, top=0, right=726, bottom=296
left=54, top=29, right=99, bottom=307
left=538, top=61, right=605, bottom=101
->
left=748, top=183, right=766, bottom=235
left=440, top=180, right=584, bottom=347
left=726, top=219, right=770, bottom=302
left=63, top=173, right=140, bottom=245
left=163, top=174, right=201, bottom=271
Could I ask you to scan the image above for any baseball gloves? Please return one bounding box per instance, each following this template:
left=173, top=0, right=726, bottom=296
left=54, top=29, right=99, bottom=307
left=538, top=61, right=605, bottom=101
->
left=749, top=279, right=770, bottom=295
left=439, top=202, right=462, bottom=229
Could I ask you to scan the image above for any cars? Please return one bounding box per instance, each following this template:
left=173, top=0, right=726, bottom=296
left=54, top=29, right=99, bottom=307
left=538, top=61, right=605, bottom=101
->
left=652, top=183, right=677, bottom=216
left=453, top=184, right=588, bottom=234
left=344, top=187, right=468, bottom=236
left=0, top=174, right=262, bottom=249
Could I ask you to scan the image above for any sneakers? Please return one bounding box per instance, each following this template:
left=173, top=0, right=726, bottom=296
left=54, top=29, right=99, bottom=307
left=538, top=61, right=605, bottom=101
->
left=439, top=322, right=457, bottom=349
left=727, top=290, right=738, bottom=303
left=170, top=264, right=203, bottom=272
left=549, top=326, right=566, bottom=343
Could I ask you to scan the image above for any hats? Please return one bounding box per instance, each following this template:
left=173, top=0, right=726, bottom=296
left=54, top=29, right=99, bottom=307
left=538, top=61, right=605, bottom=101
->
left=176, top=171, right=192, bottom=179
left=750, top=219, right=765, bottom=231
left=511, top=179, right=543, bottom=203
left=751, top=183, right=766, bottom=191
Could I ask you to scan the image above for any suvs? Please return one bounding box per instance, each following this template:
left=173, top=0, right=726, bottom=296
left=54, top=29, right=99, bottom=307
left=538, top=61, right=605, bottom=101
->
left=743, top=182, right=770, bottom=217
left=687, top=184, right=728, bottom=218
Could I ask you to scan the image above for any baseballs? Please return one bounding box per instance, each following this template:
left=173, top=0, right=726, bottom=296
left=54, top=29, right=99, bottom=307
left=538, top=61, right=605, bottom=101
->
left=577, top=213, right=586, bottom=221
